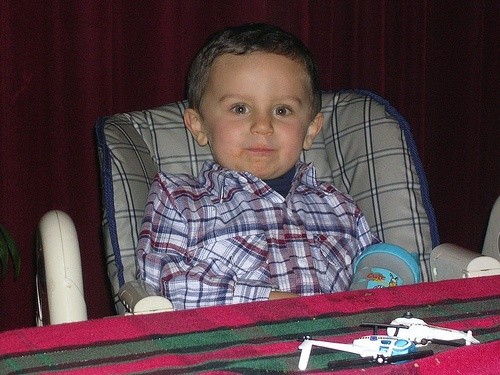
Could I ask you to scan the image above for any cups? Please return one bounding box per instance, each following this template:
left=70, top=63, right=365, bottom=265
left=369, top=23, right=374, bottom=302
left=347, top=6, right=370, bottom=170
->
left=345, top=243, right=419, bottom=291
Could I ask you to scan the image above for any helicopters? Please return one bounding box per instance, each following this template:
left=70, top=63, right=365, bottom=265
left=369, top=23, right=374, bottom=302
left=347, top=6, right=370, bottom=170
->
left=298, top=311, right=481, bottom=371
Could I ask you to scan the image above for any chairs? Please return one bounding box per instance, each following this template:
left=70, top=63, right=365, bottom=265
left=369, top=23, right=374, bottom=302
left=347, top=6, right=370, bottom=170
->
left=96, top=88, right=441, bottom=315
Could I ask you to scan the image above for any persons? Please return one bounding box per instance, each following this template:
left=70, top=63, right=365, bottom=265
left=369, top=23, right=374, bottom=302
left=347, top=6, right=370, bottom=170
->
left=133, top=23, right=381, bottom=311
